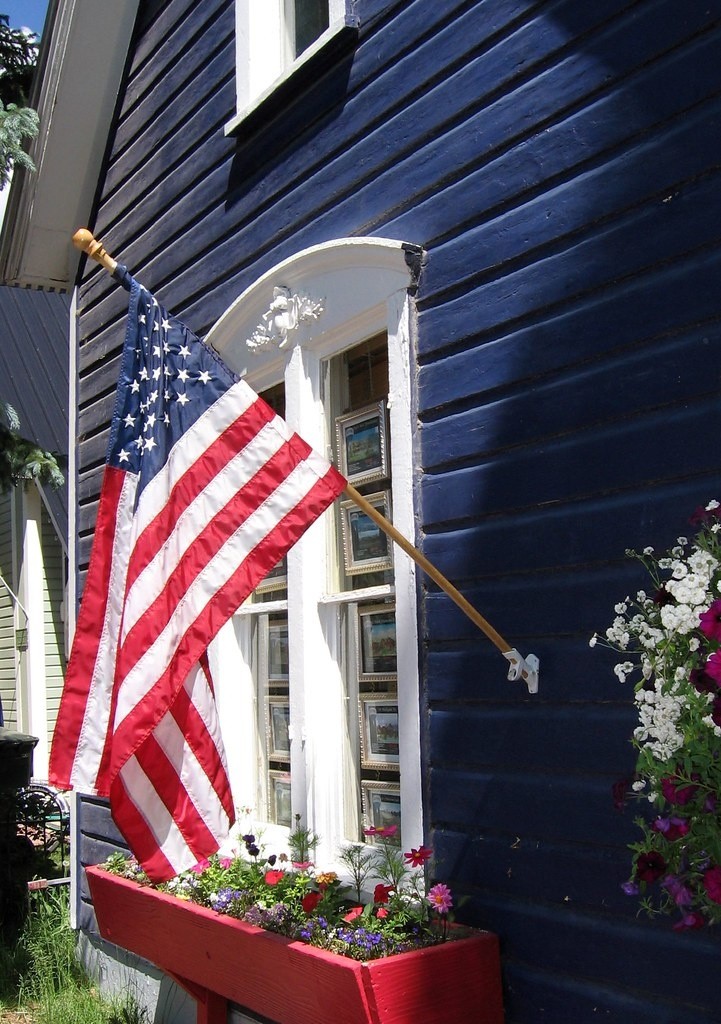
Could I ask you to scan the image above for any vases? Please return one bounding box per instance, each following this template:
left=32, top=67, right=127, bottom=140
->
left=84, top=864, right=504, bottom=1024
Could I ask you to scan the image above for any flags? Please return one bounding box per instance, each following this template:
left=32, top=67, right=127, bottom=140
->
left=45, top=263, right=348, bottom=885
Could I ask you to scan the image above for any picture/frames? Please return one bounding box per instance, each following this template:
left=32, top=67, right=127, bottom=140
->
left=361, top=780, right=403, bottom=847
left=355, top=602, right=398, bottom=682
left=334, top=399, right=390, bottom=489
left=266, top=619, right=289, bottom=689
left=255, top=553, right=287, bottom=594
left=263, top=695, right=291, bottom=764
left=358, top=692, right=400, bottom=772
left=341, top=490, right=395, bottom=577
left=268, top=770, right=291, bottom=829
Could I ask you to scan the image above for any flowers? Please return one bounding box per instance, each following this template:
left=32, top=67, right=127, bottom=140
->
left=589, top=501, right=721, bottom=931
left=96, top=803, right=470, bottom=961
